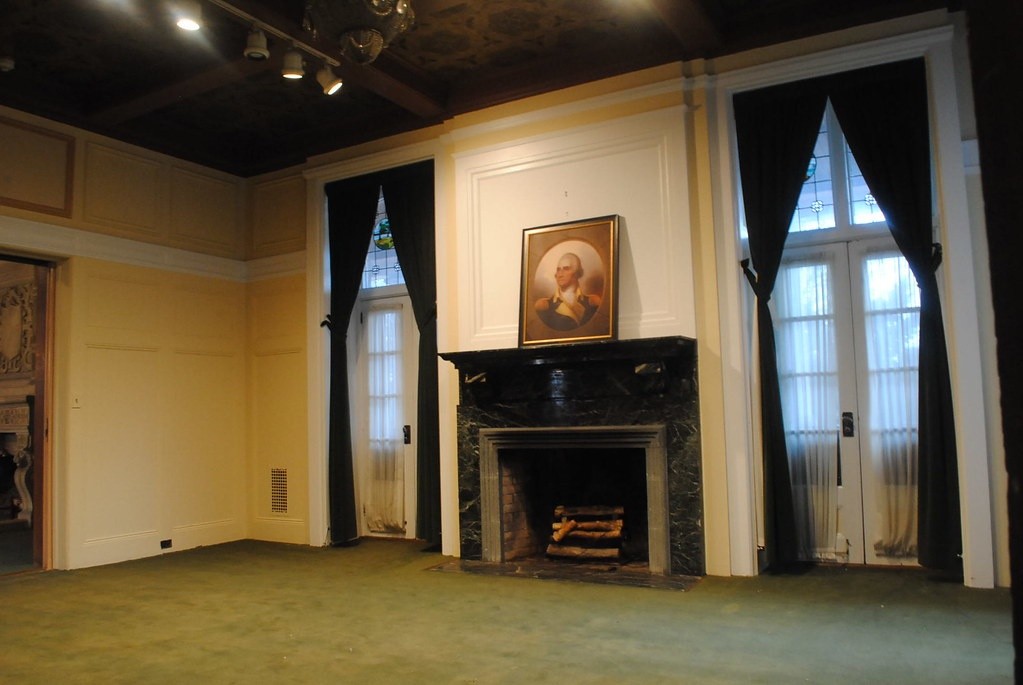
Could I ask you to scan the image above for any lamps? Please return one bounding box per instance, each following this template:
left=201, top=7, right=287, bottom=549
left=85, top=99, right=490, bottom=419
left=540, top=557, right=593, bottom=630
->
left=165, top=0, right=415, bottom=98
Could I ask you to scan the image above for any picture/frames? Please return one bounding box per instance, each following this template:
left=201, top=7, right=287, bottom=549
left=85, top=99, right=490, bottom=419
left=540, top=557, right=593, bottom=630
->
left=517, top=214, right=619, bottom=349
